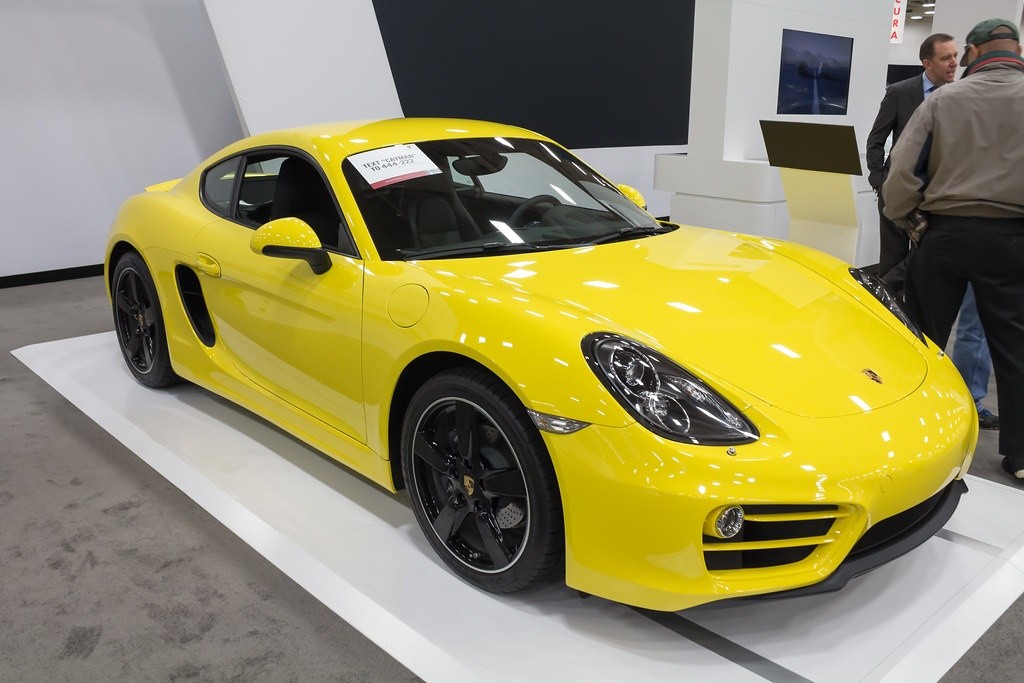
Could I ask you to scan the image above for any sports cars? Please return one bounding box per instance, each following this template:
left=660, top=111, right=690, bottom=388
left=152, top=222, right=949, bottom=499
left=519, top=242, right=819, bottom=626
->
left=103, top=118, right=981, bottom=612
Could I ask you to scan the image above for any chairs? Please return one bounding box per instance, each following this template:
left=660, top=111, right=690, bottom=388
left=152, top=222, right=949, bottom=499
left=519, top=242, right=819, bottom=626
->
left=270, top=158, right=339, bottom=248
left=400, top=164, right=484, bottom=250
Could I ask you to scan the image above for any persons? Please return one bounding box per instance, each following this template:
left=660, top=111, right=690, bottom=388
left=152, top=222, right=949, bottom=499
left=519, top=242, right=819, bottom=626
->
left=866, top=33, right=998, bottom=430
left=882, top=18, right=1024, bottom=488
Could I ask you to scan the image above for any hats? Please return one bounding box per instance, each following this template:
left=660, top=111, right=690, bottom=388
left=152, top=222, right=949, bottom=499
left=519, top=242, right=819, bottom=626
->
left=959, top=18, right=1020, bottom=68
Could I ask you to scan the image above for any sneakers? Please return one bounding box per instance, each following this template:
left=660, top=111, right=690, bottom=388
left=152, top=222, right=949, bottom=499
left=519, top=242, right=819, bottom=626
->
left=978, top=409, right=1000, bottom=429
left=1002, top=456, right=1024, bottom=479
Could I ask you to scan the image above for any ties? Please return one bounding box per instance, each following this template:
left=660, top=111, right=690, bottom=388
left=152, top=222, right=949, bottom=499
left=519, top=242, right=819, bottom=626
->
left=930, top=86, right=938, bottom=93
